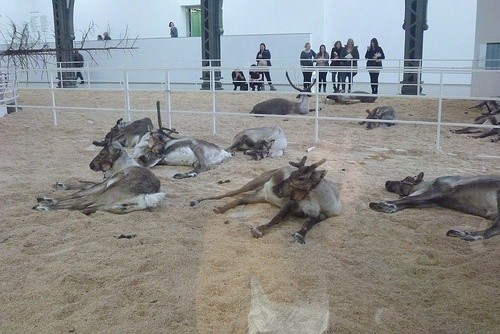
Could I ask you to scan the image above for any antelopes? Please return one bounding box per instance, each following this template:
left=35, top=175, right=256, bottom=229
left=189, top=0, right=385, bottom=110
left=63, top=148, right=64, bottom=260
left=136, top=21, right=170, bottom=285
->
left=248, top=71, right=323, bottom=115
left=359, top=106, right=396, bottom=130
left=225, top=126, right=287, bottom=161
left=328, top=83, right=380, bottom=105
left=189, top=157, right=343, bottom=244
left=368, top=171, right=500, bottom=241
left=451, top=101, right=500, bottom=142
left=33, top=101, right=233, bottom=216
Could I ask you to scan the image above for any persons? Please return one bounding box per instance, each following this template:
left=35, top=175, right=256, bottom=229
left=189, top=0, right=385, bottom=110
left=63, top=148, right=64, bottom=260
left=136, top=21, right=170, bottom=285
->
left=299, top=38, right=359, bottom=93
left=232, top=43, right=277, bottom=91
left=74, top=50, right=84, bottom=84
left=97, top=32, right=111, bottom=40
left=365, top=37, right=385, bottom=94
left=169, top=21, right=178, bottom=38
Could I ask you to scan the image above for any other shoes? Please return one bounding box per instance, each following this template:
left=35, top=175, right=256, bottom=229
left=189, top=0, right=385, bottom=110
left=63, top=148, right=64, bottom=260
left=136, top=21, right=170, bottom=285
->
left=340, top=89, right=345, bottom=92
left=348, top=88, right=351, bottom=92
left=252, top=86, right=256, bottom=90
left=257, top=87, right=260, bottom=91
left=270, top=83, right=276, bottom=90
left=260, top=83, right=265, bottom=90
left=79, top=81, right=84, bottom=84
